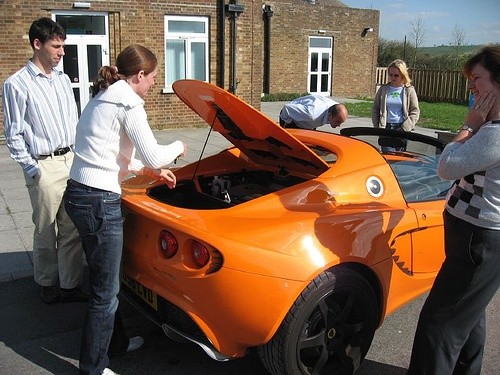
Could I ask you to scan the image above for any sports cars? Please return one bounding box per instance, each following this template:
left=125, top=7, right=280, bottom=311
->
left=120, top=79, right=450, bottom=375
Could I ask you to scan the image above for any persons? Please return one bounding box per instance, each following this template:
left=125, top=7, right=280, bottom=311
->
left=279, top=95, right=348, bottom=131
left=3, top=17, right=90, bottom=305
left=407, top=43, right=500, bottom=375
left=372, top=59, right=420, bottom=152
left=63, top=44, right=188, bottom=375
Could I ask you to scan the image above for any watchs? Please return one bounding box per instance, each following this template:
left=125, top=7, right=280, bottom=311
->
left=460, top=125, right=474, bottom=132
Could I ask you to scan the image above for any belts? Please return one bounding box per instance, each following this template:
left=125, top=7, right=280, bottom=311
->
left=69, top=179, right=111, bottom=192
left=45, top=144, right=74, bottom=157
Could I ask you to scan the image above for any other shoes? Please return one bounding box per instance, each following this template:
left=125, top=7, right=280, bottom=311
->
left=127, top=335, right=143, bottom=352
left=61, top=288, right=90, bottom=302
left=41, top=284, right=58, bottom=303
left=102, top=369, right=117, bottom=375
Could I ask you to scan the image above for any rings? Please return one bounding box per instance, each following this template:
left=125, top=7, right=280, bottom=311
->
left=475, top=105, right=480, bottom=109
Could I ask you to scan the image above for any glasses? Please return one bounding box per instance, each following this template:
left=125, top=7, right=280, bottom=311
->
left=389, top=74, right=403, bottom=78
